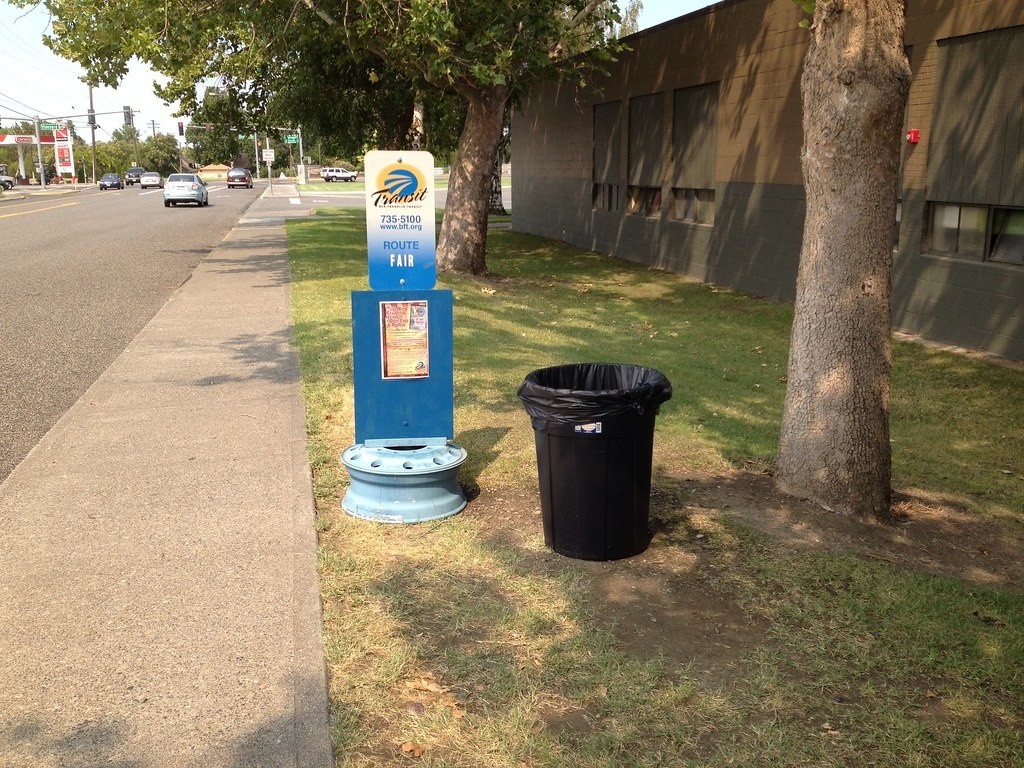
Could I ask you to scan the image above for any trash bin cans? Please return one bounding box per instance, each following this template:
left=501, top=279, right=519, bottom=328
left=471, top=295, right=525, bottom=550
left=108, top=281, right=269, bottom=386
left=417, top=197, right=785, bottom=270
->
left=523, top=362, right=669, bottom=561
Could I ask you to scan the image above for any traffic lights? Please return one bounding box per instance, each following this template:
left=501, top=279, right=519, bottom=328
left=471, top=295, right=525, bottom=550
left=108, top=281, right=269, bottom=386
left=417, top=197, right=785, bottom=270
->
left=178, top=122, right=184, bottom=136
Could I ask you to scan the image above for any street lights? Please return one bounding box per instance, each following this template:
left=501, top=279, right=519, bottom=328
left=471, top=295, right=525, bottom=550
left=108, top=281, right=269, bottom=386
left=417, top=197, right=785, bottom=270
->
left=209, top=93, right=259, bottom=180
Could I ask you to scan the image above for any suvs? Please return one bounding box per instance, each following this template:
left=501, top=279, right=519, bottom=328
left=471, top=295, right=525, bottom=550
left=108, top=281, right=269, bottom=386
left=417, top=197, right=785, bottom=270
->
left=123, top=167, right=146, bottom=186
left=320, top=167, right=358, bottom=182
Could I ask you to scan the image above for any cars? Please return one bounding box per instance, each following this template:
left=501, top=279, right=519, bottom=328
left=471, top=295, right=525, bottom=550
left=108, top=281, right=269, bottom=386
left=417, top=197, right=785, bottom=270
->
left=99, top=173, right=125, bottom=191
left=226, top=167, right=254, bottom=190
left=0, top=180, right=8, bottom=192
left=140, top=171, right=165, bottom=190
left=163, top=173, right=208, bottom=208
left=0, top=176, right=16, bottom=190
